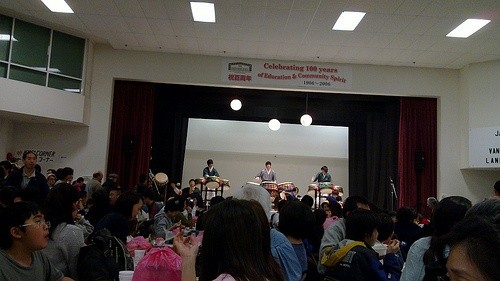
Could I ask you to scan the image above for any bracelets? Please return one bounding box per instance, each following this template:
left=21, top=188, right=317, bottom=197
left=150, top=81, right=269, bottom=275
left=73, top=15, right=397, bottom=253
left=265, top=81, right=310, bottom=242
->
left=172, top=226, right=176, bottom=229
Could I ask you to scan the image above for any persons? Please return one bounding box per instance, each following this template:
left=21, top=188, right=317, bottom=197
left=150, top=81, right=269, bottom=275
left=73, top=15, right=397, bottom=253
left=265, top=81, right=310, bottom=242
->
left=0, top=150, right=500, bottom=281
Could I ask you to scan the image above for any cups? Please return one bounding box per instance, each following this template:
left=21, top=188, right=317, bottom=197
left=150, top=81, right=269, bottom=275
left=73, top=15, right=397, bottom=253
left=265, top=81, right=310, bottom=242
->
left=118, top=271, right=134, bottom=281
left=134, top=250, right=147, bottom=268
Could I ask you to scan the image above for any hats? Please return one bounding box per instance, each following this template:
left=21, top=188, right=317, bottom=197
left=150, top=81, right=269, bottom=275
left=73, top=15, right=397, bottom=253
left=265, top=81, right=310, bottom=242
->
left=46, top=170, right=56, bottom=179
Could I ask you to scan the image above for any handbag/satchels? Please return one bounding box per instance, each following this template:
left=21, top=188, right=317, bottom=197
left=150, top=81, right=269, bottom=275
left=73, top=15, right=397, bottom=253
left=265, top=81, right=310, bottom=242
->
left=132, top=247, right=183, bottom=281
left=73, top=228, right=124, bottom=281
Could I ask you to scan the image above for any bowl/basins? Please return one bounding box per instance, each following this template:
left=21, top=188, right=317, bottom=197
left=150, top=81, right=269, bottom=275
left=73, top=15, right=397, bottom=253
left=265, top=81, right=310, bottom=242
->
left=372, top=244, right=387, bottom=255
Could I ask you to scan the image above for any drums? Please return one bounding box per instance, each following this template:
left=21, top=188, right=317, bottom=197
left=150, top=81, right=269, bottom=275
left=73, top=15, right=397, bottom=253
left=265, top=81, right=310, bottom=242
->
left=155, top=173, right=168, bottom=185
left=308, top=184, right=319, bottom=195
left=333, top=186, right=344, bottom=196
left=206, top=176, right=220, bottom=189
left=194, top=178, right=205, bottom=190
left=218, top=180, right=230, bottom=191
left=278, top=182, right=295, bottom=194
left=246, top=182, right=260, bottom=188
left=261, top=182, right=278, bottom=191
left=319, top=182, right=332, bottom=194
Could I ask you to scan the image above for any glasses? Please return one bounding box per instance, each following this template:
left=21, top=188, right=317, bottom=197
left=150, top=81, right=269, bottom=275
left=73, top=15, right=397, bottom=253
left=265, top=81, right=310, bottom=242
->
left=18, top=217, right=51, bottom=229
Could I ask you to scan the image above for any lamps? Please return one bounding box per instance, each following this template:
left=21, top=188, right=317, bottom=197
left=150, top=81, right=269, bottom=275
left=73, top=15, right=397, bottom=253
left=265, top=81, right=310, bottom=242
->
left=300, top=93, right=312, bottom=127
